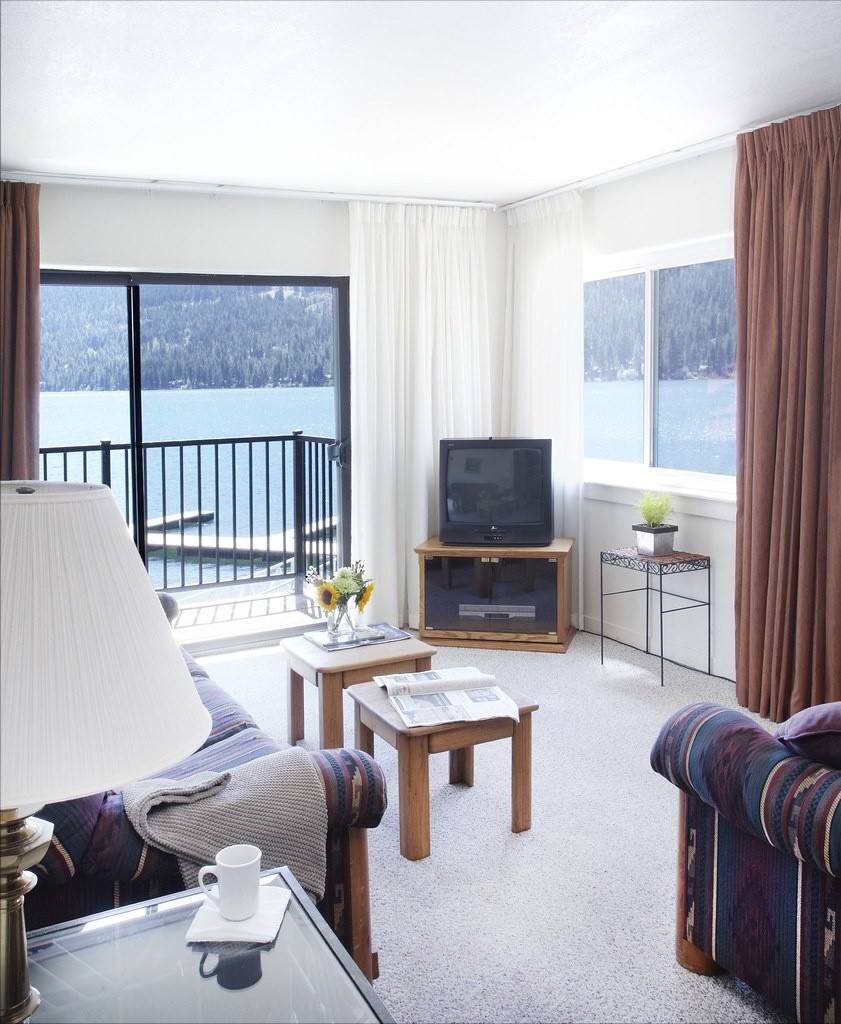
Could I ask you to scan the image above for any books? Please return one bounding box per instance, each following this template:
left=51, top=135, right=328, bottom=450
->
left=305, top=622, right=415, bottom=651
left=372, top=667, right=520, bottom=728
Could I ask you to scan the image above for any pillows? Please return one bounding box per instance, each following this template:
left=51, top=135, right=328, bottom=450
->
left=772, top=701, right=841, bottom=769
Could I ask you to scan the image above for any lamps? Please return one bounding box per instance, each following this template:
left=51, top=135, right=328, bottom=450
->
left=0, top=479, right=212, bottom=1024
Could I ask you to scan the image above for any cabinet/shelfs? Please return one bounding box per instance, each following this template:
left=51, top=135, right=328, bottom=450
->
left=413, top=534, right=578, bottom=653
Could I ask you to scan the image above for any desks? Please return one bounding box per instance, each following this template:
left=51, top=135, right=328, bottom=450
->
left=347, top=666, right=539, bottom=861
left=0, top=864, right=400, bottom=1024
left=279, top=620, right=437, bottom=750
left=599, top=545, right=712, bottom=686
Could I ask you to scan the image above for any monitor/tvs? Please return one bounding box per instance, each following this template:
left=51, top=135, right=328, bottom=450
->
left=438, top=438, right=554, bottom=547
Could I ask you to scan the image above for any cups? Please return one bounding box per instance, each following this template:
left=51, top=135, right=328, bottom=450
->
left=198, top=845, right=262, bottom=921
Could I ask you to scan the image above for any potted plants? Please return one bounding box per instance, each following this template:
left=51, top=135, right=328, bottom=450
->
left=631, top=490, right=679, bottom=558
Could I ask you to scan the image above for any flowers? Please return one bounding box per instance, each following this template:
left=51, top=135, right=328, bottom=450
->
left=305, top=559, right=376, bottom=631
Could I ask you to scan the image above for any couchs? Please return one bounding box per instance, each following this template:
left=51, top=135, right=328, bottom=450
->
left=25, top=591, right=390, bottom=989
left=649, top=700, right=841, bottom=1022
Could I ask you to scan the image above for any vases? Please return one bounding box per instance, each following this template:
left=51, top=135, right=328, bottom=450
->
left=328, top=595, right=355, bottom=634
